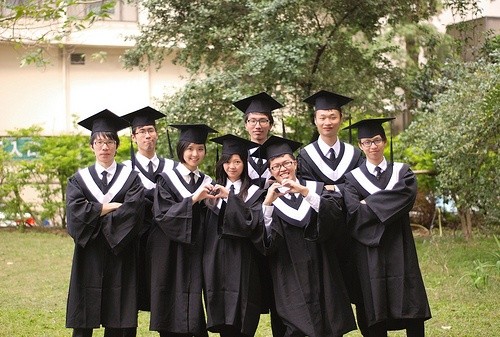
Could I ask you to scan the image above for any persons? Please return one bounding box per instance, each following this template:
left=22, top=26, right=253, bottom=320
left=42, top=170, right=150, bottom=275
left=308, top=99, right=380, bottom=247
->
left=204, top=135, right=269, bottom=337
left=340, top=117, right=433, bottom=337
left=248, top=137, right=357, bottom=337
left=65, top=109, right=144, bottom=337
left=232, top=92, right=285, bottom=314
left=148, top=123, right=215, bottom=337
left=119, top=106, right=181, bottom=312
left=296, top=90, right=363, bottom=303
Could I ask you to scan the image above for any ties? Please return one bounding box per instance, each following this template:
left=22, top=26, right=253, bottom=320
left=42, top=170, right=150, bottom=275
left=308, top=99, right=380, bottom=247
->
left=148, top=161, right=153, bottom=174
left=101, top=171, right=108, bottom=185
left=289, top=193, right=297, bottom=205
left=330, top=148, right=335, bottom=162
left=229, top=184, right=235, bottom=194
left=375, top=167, right=383, bottom=185
left=188, top=173, right=196, bottom=185
left=257, top=158, right=263, bottom=168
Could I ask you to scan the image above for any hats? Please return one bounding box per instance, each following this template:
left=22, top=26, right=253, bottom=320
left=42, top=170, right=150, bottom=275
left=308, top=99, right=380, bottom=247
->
left=302, top=90, right=352, bottom=143
left=232, top=92, right=286, bottom=139
left=342, top=117, right=396, bottom=173
left=119, top=106, right=173, bottom=158
left=76, top=109, right=135, bottom=170
left=167, top=124, right=219, bottom=181
left=250, top=135, right=304, bottom=186
left=209, top=134, right=262, bottom=190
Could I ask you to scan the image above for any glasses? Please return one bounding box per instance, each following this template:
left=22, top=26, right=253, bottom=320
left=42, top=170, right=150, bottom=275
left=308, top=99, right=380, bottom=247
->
left=94, top=138, right=116, bottom=147
left=270, top=161, right=294, bottom=171
left=247, top=118, right=269, bottom=126
left=361, top=139, right=383, bottom=147
left=134, top=130, right=156, bottom=136
